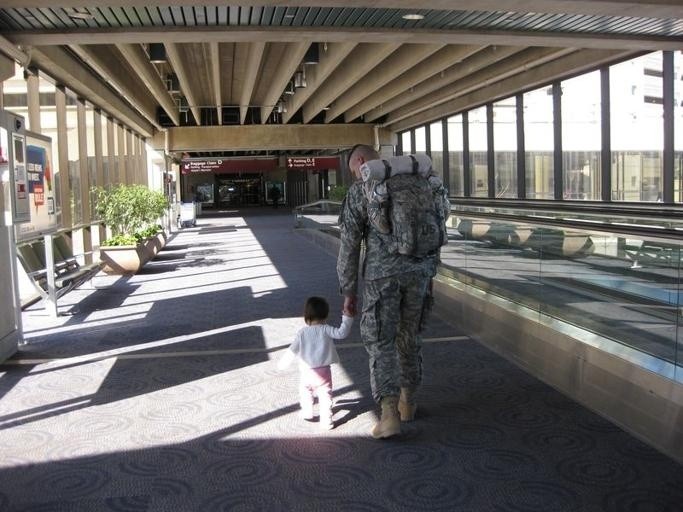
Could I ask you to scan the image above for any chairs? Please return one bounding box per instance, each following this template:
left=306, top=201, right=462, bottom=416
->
left=15, top=231, right=107, bottom=318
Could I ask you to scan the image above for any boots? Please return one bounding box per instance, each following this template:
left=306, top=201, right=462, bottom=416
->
left=371, top=393, right=401, bottom=439
left=397, top=385, right=418, bottom=421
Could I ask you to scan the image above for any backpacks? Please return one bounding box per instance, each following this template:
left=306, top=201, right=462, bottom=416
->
left=364, top=173, right=449, bottom=259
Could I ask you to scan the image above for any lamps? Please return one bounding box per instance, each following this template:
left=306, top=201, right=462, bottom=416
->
left=275, top=42, right=318, bottom=114
left=148, top=43, right=188, bottom=114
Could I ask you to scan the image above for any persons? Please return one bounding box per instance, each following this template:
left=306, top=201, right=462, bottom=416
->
left=288, top=296, right=356, bottom=431
left=269, top=183, right=280, bottom=209
left=334, top=142, right=442, bottom=441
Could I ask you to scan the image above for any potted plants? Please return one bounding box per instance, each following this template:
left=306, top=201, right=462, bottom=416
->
left=89, top=182, right=171, bottom=276
left=326, top=184, right=350, bottom=215
